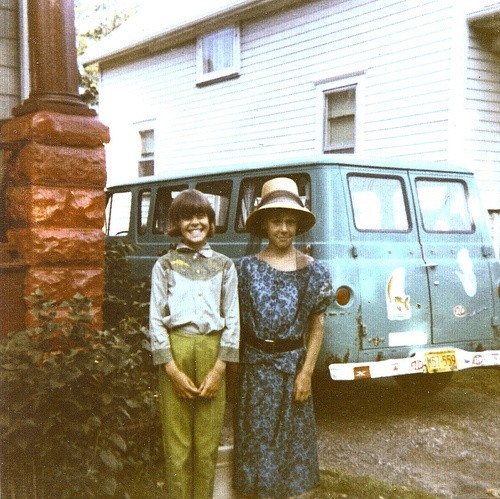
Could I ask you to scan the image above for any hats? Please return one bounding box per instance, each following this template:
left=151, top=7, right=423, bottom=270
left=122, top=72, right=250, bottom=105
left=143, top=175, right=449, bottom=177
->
left=243, top=177, right=316, bottom=238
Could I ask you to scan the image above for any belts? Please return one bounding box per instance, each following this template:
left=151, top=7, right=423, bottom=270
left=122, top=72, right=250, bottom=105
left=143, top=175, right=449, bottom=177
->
left=242, top=331, right=304, bottom=355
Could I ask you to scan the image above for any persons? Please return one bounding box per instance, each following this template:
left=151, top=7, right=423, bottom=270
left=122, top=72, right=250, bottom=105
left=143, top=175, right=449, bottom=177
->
left=149, top=188, right=242, bottom=499
left=235, top=176, right=336, bottom=499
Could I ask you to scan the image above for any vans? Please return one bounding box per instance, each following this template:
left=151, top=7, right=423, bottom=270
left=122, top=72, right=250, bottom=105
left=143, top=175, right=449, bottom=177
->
left=103, top=153, right=500, bottom=393
